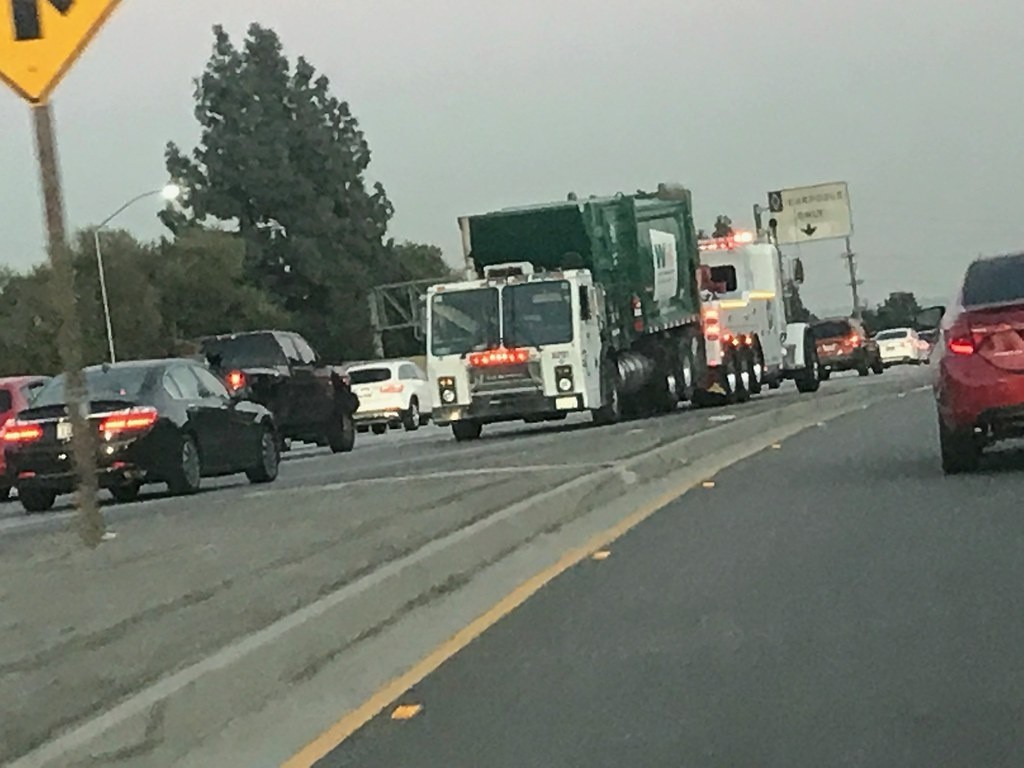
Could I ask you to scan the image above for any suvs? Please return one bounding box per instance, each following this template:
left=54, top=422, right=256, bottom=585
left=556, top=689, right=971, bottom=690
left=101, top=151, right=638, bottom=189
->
left=342, top=361, right=432, bottom=436
left=813, top=316, right=884, bottom=380
left=1, top=376, right=56, bottom=503
left=175, top=332, right=359, bottom=454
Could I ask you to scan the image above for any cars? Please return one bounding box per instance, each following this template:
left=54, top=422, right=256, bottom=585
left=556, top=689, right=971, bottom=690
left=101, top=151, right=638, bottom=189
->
left=911, top=250, right=1024, bottom=474
left=873, top=328, right=931, bottom=368
left=5, top=357, right=282, bottom=514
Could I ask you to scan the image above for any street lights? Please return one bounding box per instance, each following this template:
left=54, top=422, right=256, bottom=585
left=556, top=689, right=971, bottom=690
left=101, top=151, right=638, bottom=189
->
left=92, top=181, right=181, bottom=364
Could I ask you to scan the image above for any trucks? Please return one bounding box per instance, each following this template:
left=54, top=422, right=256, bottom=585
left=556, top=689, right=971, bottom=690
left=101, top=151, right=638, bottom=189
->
left=697, top=231, right=820, bottom=404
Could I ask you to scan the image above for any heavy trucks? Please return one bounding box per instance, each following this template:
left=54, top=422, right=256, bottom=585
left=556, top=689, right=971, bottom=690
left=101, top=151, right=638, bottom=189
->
left=411, top=184, right=705, bottom=441
